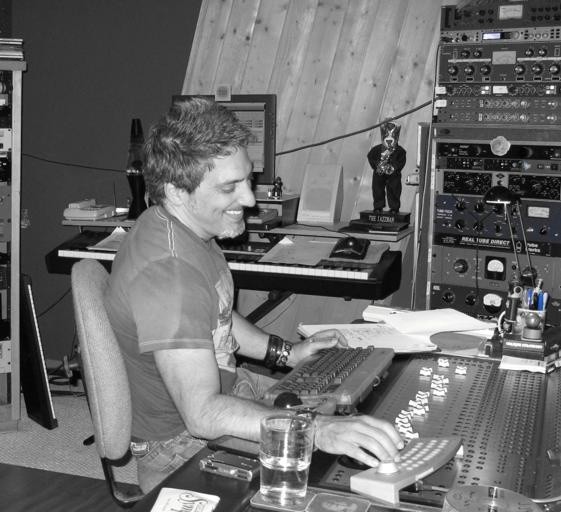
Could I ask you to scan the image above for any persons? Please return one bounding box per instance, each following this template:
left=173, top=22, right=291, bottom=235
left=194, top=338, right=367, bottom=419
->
left=103, top=96, right=406, bottom=499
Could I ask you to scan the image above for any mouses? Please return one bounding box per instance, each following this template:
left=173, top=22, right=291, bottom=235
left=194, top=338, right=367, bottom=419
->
left=275, top=392, right=302, bottom=408
left=336, top=235, right=362, bottom=254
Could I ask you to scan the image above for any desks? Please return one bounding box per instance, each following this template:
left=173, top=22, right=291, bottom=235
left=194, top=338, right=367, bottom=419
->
left=128, top=318, right=561, bottom=512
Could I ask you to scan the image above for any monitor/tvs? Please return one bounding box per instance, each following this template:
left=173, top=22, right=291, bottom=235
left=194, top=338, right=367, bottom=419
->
left=172, top=92, right=278, bottom=186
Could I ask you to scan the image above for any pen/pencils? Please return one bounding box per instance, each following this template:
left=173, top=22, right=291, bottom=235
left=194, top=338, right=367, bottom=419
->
left=522, top=278, right=548, bottom=311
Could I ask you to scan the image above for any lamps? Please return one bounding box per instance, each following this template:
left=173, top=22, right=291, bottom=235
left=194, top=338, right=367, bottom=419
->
left=484, top=184, right=537, bottom=301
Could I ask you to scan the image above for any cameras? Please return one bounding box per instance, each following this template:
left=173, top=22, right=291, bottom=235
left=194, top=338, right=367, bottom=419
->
left=198, top=452, right=260, bottom=482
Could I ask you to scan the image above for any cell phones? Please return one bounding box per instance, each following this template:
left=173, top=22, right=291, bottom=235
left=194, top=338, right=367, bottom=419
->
left=206, top=433, right=262, bottom=460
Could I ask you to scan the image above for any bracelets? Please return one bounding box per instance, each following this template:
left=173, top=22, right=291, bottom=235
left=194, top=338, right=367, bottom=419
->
left=263, top=333, right=285, bottom=369
left=278, top=340, right=294, bottom=365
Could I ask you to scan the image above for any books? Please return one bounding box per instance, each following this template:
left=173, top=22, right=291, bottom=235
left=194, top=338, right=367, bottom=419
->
left=295, top=306, right=493, bottom=358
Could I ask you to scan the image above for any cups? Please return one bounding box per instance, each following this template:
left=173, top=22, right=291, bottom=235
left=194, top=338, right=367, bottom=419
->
left=258, top=415, right=316, bottom=504
left=497, top=307, right=545, bottom=336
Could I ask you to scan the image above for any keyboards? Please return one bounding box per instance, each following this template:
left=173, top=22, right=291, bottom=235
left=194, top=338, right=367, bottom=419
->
left=246, top=207, right=278, bottom=225
left=262, top=344, right=395, bottom=414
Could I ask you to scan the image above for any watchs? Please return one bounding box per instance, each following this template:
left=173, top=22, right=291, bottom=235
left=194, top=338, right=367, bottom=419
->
left=289, top=409, right=319, bottom=460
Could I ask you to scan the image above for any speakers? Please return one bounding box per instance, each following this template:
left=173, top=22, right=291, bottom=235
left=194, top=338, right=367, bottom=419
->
left=297, top=163, right=346, bottom=227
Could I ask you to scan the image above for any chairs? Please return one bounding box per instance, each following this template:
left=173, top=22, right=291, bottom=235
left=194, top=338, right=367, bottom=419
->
left=70, top=258, right=141, bottom=507
